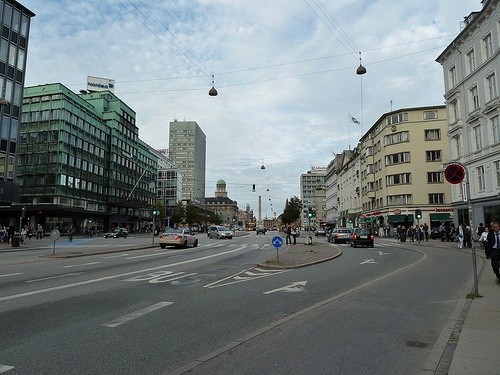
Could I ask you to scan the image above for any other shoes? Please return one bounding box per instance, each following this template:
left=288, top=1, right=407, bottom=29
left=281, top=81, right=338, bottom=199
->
left=288, top=244, right=290, bottom=246
left=496, top=279, right=500, bottom=284
left=458, top=246, right=463, bottom=248
left=487, top=256, right=489, bottom=259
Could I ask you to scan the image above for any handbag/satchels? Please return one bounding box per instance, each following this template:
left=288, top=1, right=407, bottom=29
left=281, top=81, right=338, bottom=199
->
left=291, top=231, right=297, bottom=234
left=454, top=236, right=460, bottom=243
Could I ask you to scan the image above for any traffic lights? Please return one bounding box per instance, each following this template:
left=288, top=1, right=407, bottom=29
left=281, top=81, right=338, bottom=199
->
left=415, top=209, right=422, bottom=219
left=88, top=217, right=93, bottom=224
left=151, top=206, right=157, bottom=216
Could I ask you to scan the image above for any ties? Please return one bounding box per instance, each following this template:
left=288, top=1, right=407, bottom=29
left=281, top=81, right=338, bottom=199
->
left=496, top=233, right=499, bottom=248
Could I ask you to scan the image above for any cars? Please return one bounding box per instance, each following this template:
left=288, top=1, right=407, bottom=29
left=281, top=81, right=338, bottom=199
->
left=104, top=227, right=128, bottom=239
left=314, top=228, right=326, bottom=236
left=329, top=228, right=352, bottom=244
left=225, top=224, right=301, bottom=237
left=157, top=226, right=199, bottom=249
left=0, top=224, right=25, bottom=245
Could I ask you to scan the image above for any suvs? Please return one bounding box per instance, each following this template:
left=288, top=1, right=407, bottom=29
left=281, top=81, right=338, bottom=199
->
left=207, top=225, right=234, bottom=240
left=348, top=227, right=375, bottom=248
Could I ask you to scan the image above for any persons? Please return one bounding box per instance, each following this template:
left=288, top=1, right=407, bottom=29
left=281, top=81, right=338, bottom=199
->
left=396, top=223, right=429, bottom=242
left=181, top=225, right=187, bottom=234
left=67, top=225, right=74, bottom=243
left=0, top=223, right=43, bottom=244
left=256, top=227, right=265, bottom=236
left=477, top=223, right=500, bottom=285
left=85, top=225, right=95, bottom=239
left=326, top=226, right=332, bottom=242
left=307, top=227, right=315, bottom=233
left=440, top=223, right=472, bottom=249
left=285, top=223, right=297, bottom=246
left=378, top=226, right=390, bottom=238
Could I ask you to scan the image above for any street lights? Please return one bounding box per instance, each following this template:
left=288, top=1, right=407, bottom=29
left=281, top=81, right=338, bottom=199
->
left=307, top=201, right=312, bottom=235
